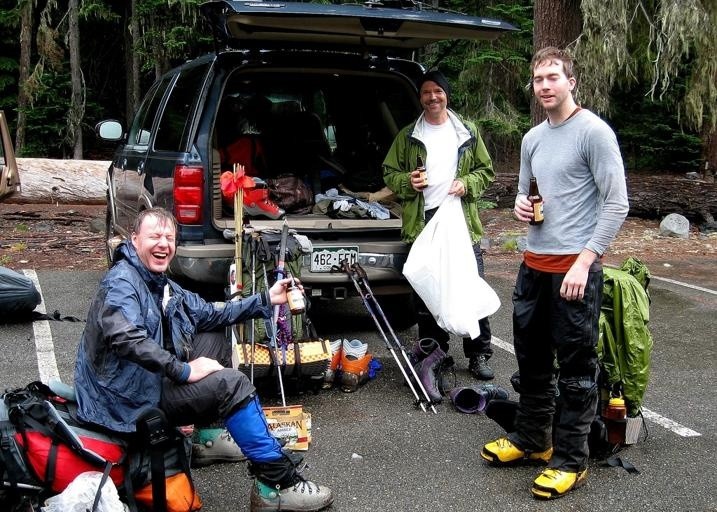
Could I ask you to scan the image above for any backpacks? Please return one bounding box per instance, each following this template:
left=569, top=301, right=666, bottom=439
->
left=222, top=257, right=328, bottom=391
left=591, top=260, right=653, bottom=457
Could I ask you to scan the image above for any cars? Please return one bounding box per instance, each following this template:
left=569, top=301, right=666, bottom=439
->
left=93, top=2, right=520, bottom=333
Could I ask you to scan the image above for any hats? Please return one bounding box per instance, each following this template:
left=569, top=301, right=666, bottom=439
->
left=418, top=70, right=451, bottom=106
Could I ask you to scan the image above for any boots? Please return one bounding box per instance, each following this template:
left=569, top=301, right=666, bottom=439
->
left=412, top=339, right=454, bottom=404
left=450, top=385, right=509, bottom=416
left=338, top=338, right=369, bottom=392
left=323, top=339, right=340, bottom=389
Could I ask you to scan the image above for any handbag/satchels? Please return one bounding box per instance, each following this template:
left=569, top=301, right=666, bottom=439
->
left=0, top=378, right=203, bottom=512
left=267, top=171, right=314, bottom=216
left=0, top=265, right=41, bottom=317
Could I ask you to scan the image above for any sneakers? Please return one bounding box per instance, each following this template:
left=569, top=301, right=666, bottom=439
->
left=480, top=436, right=554, bottom=466
left=469, top=355, right=493, bottom=378
left=251, top=477, right=334, bottom=510
left=531, top=469, right=588, bottom=499
left=192, top=429, right=250, bottom=465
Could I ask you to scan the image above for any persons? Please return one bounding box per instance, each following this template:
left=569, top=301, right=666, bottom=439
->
left=379, top=70, right=495, bottom=381
left=71, top=204, right=336, bottom=510
left=479, top=48, right=629, bottom=501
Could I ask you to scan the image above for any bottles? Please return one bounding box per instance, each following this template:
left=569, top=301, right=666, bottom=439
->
left=606, top=399, right=626, bottom=444
left=286, top=269, right=305, bottom=318
left=414, top=155, right=428, bottom=188
left=526, top=177, right=545, bottom=225
